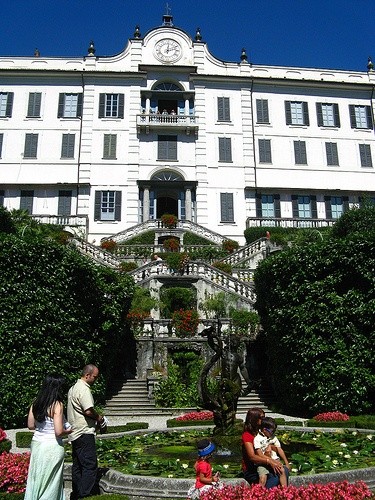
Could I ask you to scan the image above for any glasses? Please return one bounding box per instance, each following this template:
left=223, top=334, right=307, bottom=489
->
left=266, top=428, right=276, bottom=434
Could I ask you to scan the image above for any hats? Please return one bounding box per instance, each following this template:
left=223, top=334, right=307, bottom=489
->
left=196, top=439, right=215, bottom=457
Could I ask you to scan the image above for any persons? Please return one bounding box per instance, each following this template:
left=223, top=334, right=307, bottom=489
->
left=67, top=364, right=107, bottom=500
left=155, top=109, right=176, bottom=123
left=253, top=416, right=292, bottom=488
left=239, top=408, right=290, bottom=488
left=201, top=319, right=253, bottom=436
left=23, top=372, right=74, bottom=500
left=187, top=440, right=221, bottom=500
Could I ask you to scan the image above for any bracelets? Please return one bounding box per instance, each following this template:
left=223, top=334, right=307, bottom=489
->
left=210, top=477, right=214, bottom=481
